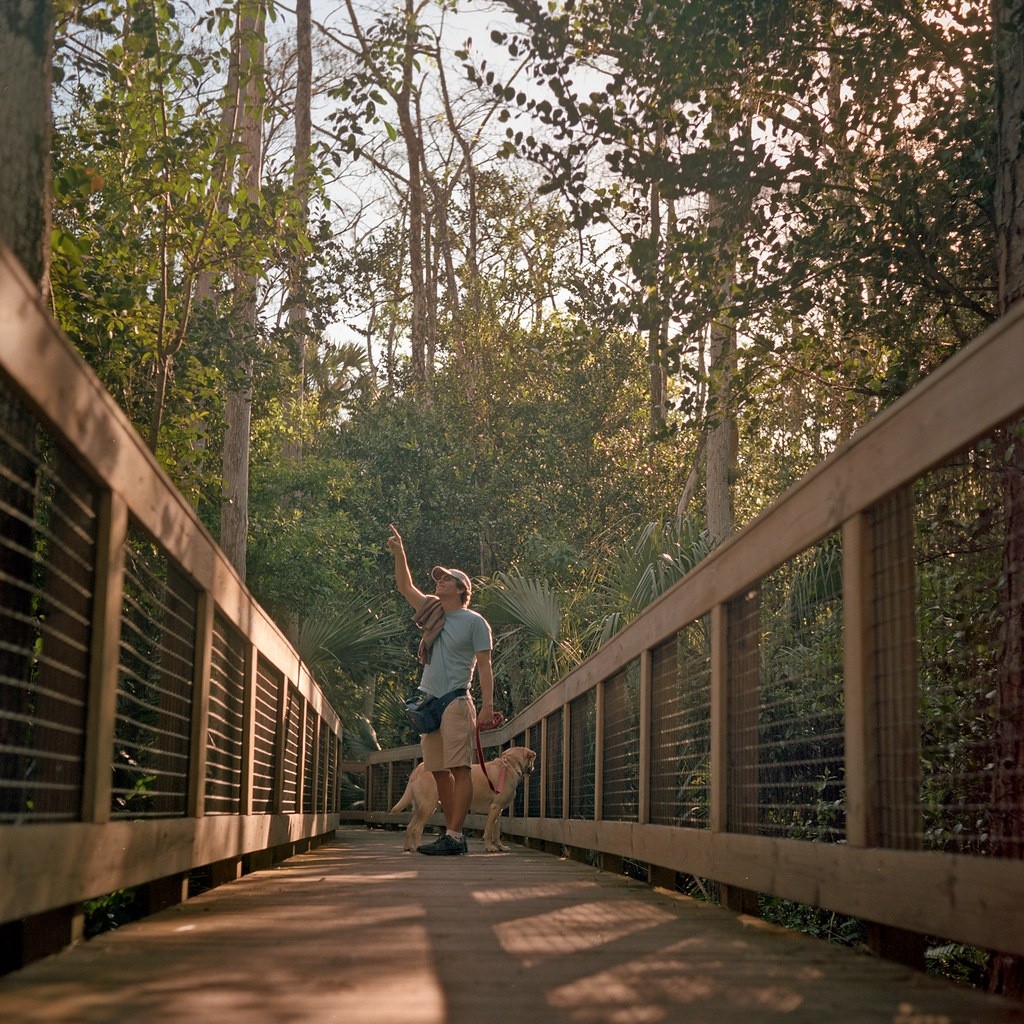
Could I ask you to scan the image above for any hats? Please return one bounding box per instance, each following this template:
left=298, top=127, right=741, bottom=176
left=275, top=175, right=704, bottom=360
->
left=431, top=565, right=471, bottom=593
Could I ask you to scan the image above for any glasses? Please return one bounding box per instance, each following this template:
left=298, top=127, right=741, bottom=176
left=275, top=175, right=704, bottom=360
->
left=436, top=576, right=456, bottom=584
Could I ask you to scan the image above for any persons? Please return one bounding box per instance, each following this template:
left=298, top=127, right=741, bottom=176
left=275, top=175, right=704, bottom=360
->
left=388, top=524, right=494, bottom=855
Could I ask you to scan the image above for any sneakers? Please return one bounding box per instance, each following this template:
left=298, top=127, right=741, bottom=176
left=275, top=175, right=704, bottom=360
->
left=417, top=834, right=469, bottom=856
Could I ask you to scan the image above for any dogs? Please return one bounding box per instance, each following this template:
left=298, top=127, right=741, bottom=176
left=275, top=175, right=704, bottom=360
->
left=390, top=746, right=537, bottom=853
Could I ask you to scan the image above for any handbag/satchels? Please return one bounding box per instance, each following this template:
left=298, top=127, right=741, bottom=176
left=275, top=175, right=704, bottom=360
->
left=404, top=688, right=468, bottom=733
